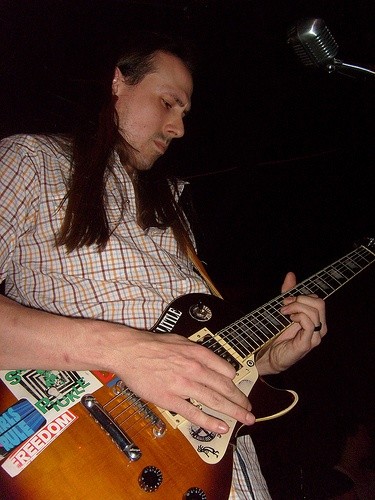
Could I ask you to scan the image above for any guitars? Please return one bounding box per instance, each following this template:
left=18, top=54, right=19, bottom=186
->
left=0, top=237, right=375, bottom=500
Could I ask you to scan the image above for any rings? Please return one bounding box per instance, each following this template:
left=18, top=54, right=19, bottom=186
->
left=314, top=322, right=323, bottom=333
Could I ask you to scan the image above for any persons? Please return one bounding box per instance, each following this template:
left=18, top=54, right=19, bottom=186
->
left=0, top=40, right=328, bottom=500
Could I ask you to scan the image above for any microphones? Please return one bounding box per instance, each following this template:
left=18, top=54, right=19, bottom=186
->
left=287, top=17, right=375, bottom=87
